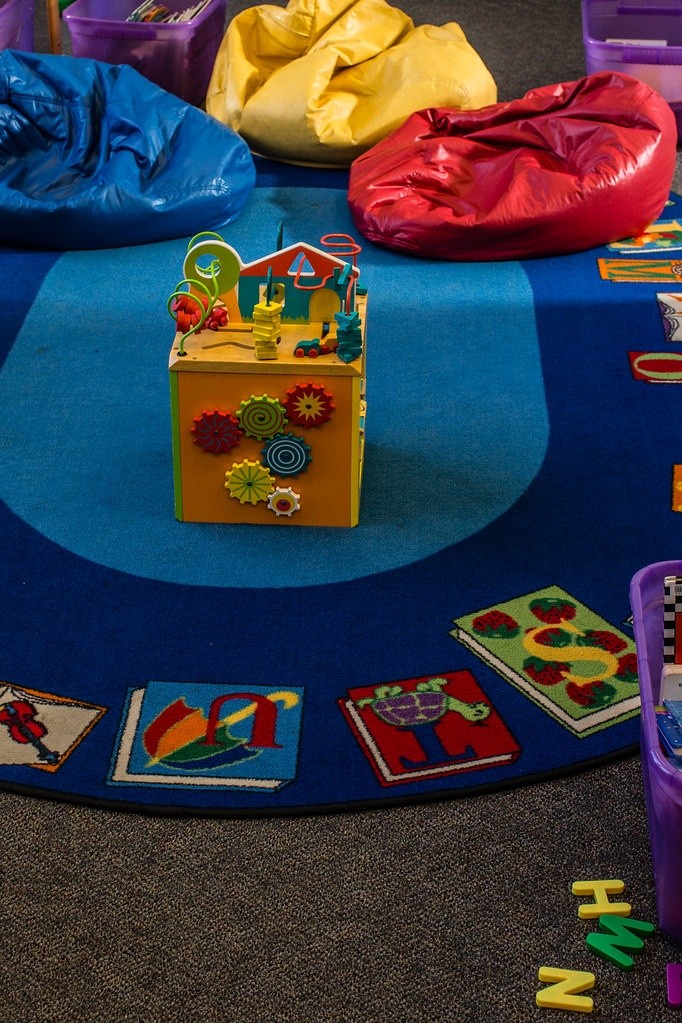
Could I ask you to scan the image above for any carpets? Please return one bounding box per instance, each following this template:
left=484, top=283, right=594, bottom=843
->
left=0, top=158, right=682, bottom=818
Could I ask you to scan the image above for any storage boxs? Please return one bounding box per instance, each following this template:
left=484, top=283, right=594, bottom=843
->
left=0, top=0, right=35, bottom=51
left=630, top=559, right=682, bottom=943
left=63, top=0, right=227, bottom=107
left=580, top=0, right=682, bottom=147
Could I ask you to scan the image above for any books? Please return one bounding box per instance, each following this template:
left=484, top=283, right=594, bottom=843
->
left=107, top=0, right=213, bottom=77
